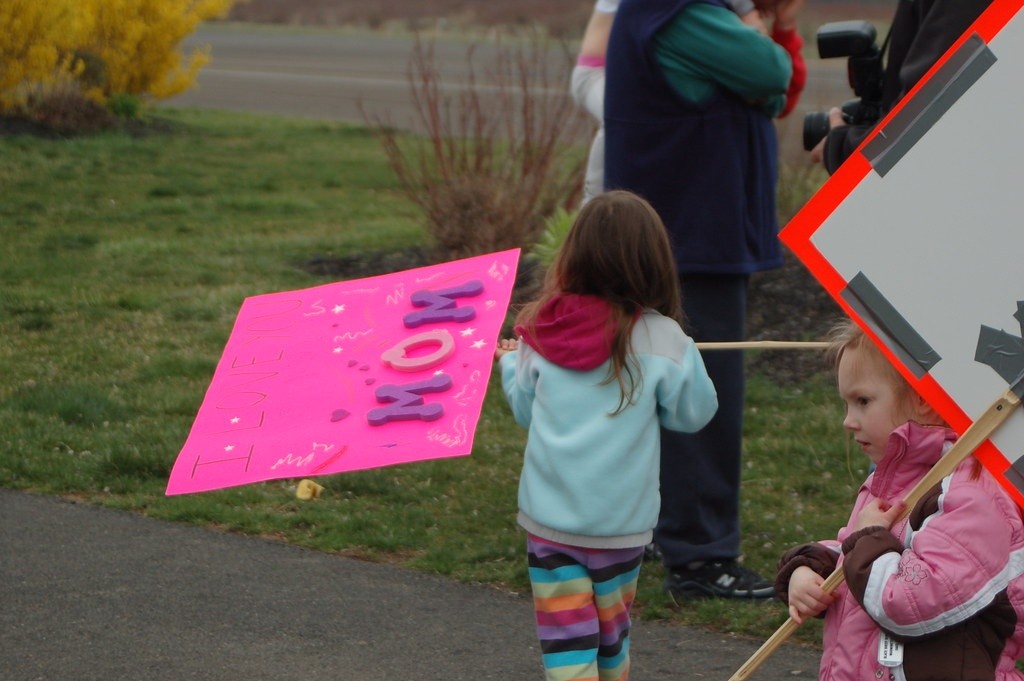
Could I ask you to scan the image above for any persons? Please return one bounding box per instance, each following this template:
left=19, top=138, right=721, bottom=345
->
left=601, top=0, right=794, bottom=603
left=490, top=189, right=720, bottom=681
left=569, top=0, right=808, bottom=209
left=803, top=0, right=993, bottom=179
left=773, top=319, right=1024, bottom=681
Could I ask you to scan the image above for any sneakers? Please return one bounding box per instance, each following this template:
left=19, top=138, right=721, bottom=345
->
left=645, top=541, right=662, bottom=558
left=662, top=558, right=774, bottom=598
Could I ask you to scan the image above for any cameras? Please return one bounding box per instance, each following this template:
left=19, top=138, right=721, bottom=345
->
left=802, top=19, right=885, bottom=153
